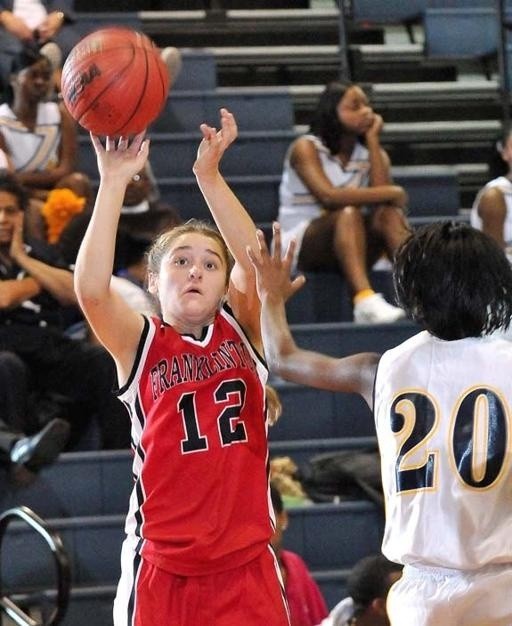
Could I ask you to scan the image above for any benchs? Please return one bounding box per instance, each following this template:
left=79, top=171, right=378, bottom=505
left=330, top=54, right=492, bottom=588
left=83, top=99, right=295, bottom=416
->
left=0, top=1, right=510, bottom=626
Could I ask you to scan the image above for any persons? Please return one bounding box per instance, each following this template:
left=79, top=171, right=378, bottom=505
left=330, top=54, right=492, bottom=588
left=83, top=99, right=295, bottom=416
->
left=244, top=221, right=512, bottom=626
left=1, top=50, right=77, bottom=182
left=268, top=479, right=329, bottom=626
left=1, top=0, right=63, bottom=50
left=2, top=417, right=73, bottom=491
left=467, top=116, right=511, bottom=264
left=1, top=182, right=114, bottom=452
left=72, top=105, right=294, bottom=626
left=272, top=76, right=417, bottom=324
left=318, top=552, right=405, bottom=626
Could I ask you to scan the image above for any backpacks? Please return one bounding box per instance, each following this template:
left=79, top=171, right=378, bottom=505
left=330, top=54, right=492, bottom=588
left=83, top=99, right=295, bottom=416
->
left=298, top=449, right=389, bottom=508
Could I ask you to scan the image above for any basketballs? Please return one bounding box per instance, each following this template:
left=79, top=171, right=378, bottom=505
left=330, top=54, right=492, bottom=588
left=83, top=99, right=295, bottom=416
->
left=60, top=26, right=167, bottom=135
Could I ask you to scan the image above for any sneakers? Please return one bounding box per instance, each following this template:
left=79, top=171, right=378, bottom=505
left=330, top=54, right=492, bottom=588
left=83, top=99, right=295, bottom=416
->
left=355, top=294, right=408, bottom=323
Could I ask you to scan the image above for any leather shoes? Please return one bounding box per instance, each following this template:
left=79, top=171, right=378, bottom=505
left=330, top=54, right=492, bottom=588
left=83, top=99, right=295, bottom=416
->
left=9, top=418, right=71, bottom=483
